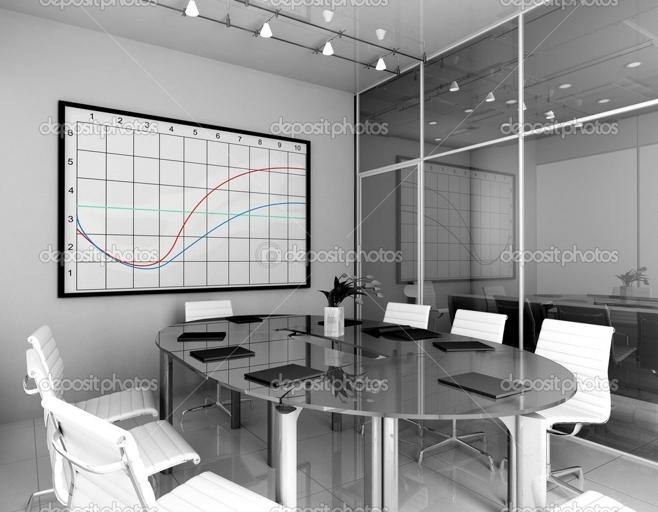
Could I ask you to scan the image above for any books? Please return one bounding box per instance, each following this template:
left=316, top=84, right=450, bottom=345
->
left=317, top=318, right=363, bottom=327
left=437, top=372, right=532, bottom=400
left=224, top=315, right=263, bottom=325
left=432, top=341, right=494, bottom=352
left=244, top=363, right=325, bottom=387
left=189, top=345, right=255, bottom=363
left=176, top=332, right=226, bottom=340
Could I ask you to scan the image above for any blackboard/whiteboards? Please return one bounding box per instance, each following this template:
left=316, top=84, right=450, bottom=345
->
left=57, top=99, right=311, bottom=298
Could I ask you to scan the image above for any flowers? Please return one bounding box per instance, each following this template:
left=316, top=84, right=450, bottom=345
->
left=318, top=272, right=384, bottom=307
